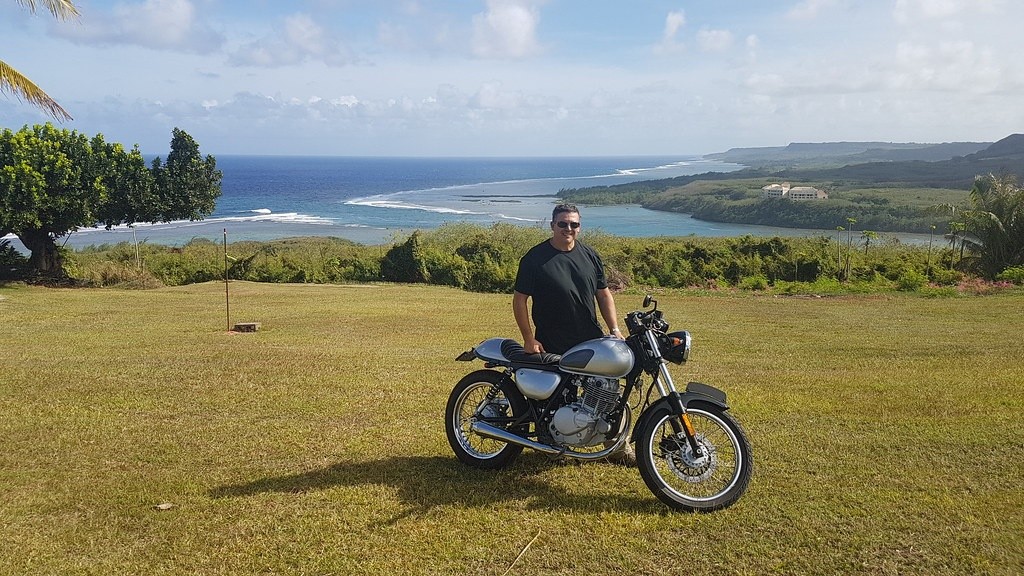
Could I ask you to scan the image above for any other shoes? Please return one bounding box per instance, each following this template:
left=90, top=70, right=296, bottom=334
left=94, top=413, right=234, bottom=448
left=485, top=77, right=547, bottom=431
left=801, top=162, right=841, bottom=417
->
left=537, top=443, right=574, bottom=463
left=605, top=448, right=637, bottom=466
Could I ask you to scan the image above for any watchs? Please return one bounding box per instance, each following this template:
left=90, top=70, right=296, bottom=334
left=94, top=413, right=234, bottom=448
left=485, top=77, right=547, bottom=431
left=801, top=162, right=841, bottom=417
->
left=610, top=328, right=620, bottom=334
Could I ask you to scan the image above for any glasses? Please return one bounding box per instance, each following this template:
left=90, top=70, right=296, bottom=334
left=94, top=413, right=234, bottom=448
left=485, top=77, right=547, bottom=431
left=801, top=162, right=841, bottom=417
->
left=553, top=221, right=580, bottom=229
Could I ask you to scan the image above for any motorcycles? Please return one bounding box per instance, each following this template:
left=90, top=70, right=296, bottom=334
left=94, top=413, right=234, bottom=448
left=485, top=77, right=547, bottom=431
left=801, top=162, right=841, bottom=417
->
left=445, top=293, right=754, bottom=512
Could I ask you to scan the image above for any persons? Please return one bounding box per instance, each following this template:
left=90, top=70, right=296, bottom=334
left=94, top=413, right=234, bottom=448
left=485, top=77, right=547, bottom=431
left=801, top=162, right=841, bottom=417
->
left=512, top=203, right=637, bottom=467
